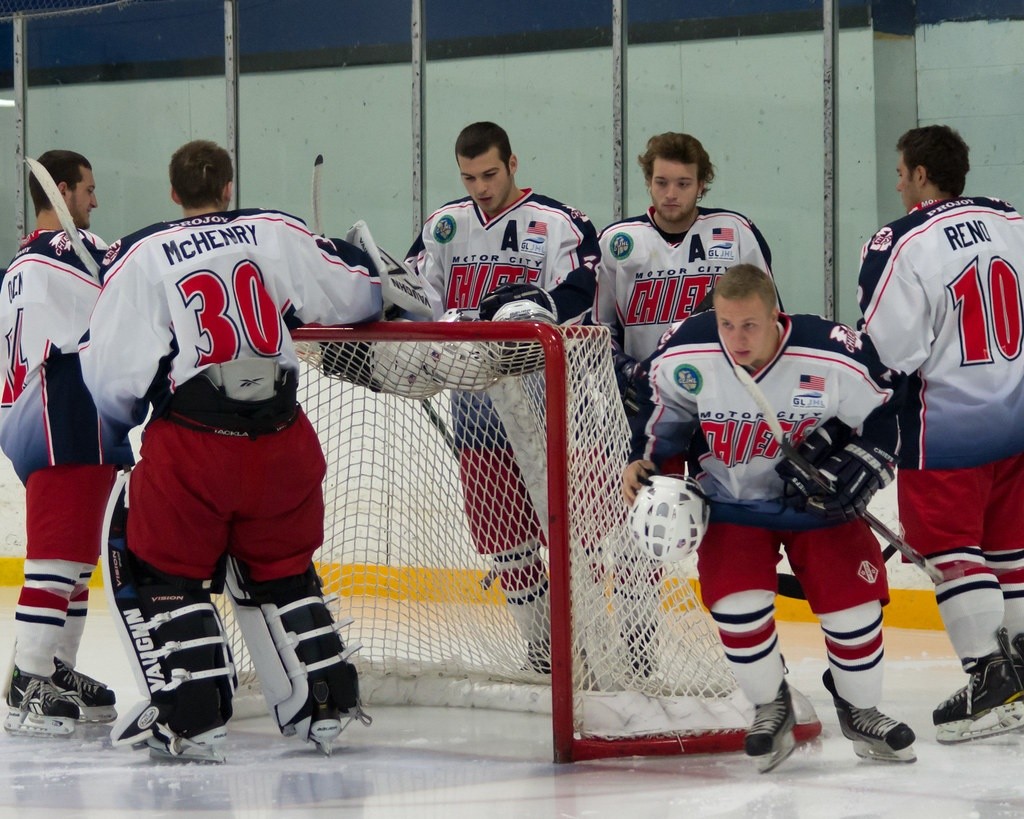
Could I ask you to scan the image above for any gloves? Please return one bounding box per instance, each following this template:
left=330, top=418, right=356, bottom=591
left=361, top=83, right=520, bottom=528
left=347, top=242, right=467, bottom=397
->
left=611, top=348, right=652, bottom=419
left=479, top=283, right=555, bottom=322
left=807, top=440, right=901, bottom=522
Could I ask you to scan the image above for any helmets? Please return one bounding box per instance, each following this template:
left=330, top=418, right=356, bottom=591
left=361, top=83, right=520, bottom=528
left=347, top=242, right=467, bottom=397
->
left=627, top=475, right=710, bottom=563
left=490, top=300, right=556, bottom=374
left=419, top=309, right=498, bottom=390
left=374, top=316, right=445, bottom=399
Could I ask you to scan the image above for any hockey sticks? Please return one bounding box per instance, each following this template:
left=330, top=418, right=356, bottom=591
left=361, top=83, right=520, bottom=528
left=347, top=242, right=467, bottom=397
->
left=733, top=365, right=944, bottom=584
left=23, top=156, right=104, bottom=287
left=312, top=154, right=460, bottom=462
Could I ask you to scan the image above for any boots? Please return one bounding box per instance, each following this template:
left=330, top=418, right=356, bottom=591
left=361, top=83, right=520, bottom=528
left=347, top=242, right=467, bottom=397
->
left=823, top=668, right=917, bottom=762
left=147, top=686, right=232, bottom=765
left=620, top=623, right=659, bottom=679
left=49, top=657, right=118, bottom=724
left=932, top=627, right=1024, bottom=746
left=744, top=655, right=797, bottom=773
left=517, top=637, right=551, bottom=675
left=2, top=665, right=80, bottom=738
left=304, top=682, right=343, bottom=757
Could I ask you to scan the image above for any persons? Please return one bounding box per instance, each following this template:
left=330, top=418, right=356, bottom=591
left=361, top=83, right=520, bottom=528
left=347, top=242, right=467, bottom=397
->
left=77, top=138, right=383, bottom=764
left=581, top=130, right=784, bottom=462
left=857, top=125, right=1024, bottom=746
left=0, top=149, right=137, bottom=739
left=400, top=119, right=661, bottom=682
left=619, top=263, right=916, bottom=774
